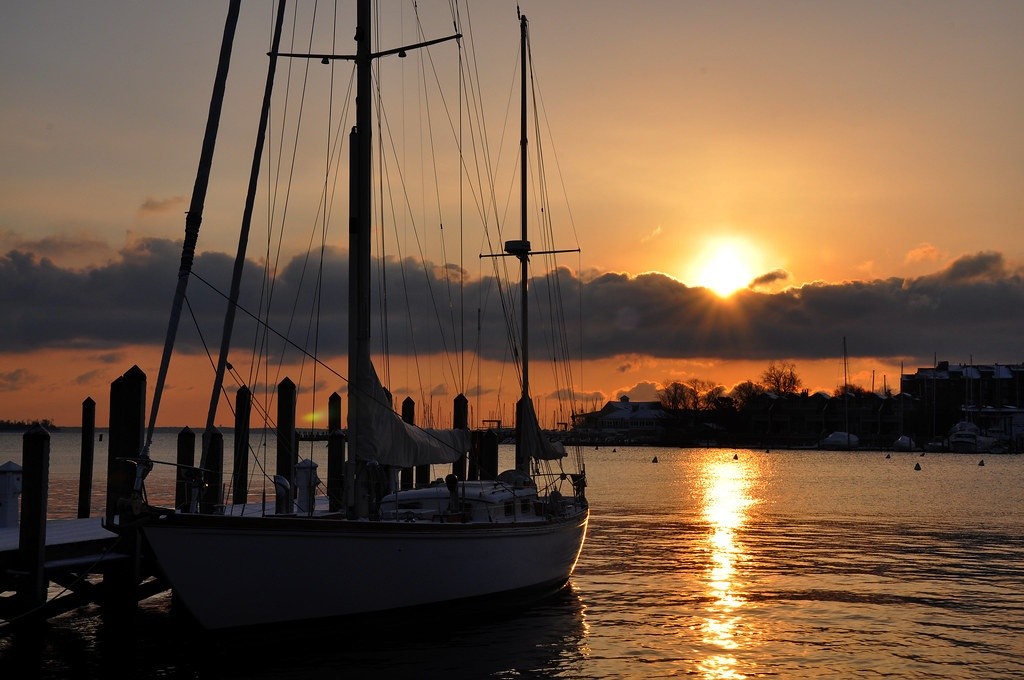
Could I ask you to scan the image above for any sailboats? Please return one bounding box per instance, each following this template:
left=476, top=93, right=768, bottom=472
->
left=130, top=0, right=591, bottom=647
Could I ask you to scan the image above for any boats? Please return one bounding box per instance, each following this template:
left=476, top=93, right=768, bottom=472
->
left=947, top=420, right=981, bottom=446
left=821, top=430, right=860, bottom=450
left=893, top=434, right=917, bottom=451
